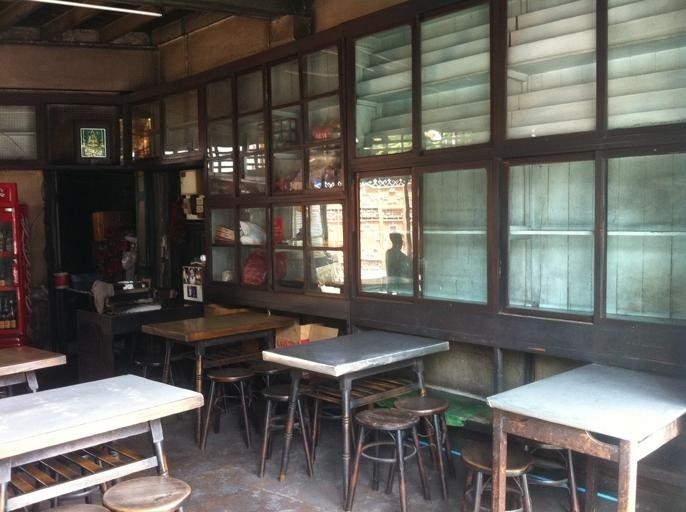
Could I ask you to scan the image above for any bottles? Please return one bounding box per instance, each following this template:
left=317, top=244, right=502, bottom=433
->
left=0, top=227, right=16, bottom=329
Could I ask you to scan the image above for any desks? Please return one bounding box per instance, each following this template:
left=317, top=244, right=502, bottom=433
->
left=141, top=313, right=299, bottom=449
left=262, top=330, right=450, bottom=510
left=1, top=373, right=207, bottom=511
left=2, top=344, right=67, bottom=400
left=486, top=362, right=686, bottom=511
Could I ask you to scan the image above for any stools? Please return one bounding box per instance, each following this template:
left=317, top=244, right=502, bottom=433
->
left=523, top=437, right=573, bottom=510
left=201, top=367, right=257, bottom=448
left=43, top=503, right=111, bottom=511
left=460, top=434, right=536, bottom=511
left=260, top=386, right=316, bottom=479
left=346, top=408, right=431, bottom=510
left=385, top=396, right=458, bottom=501
left=102, top=476, right=192, bottom=511
left=252, top=360, right=294, bottom=387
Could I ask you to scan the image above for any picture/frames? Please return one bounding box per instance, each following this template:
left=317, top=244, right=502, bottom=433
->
left=183, top=284, right=203, bottom=302
left=73, top=118, right=118, bottom=166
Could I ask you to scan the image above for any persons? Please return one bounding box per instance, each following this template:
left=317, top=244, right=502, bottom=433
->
left=386, top=234, right=410, bottom=293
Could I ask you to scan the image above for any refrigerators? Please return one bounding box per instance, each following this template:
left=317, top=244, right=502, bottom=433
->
left=0, top=181, right=34, bottom=350
